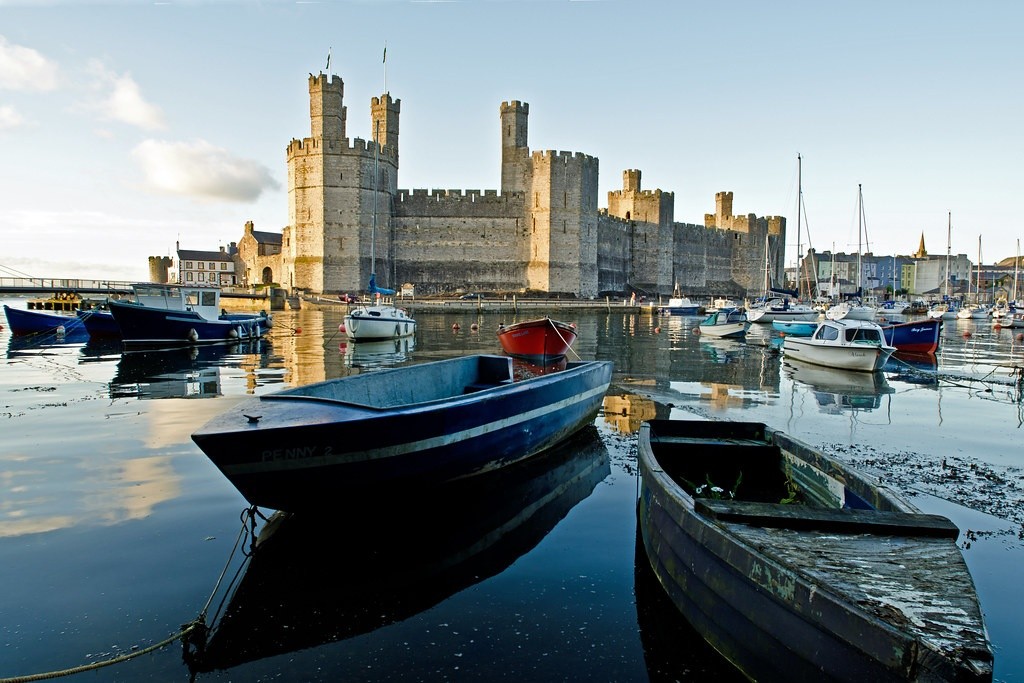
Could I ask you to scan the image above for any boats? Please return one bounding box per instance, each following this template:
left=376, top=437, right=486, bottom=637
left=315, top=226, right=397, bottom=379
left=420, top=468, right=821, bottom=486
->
left=75, top=308, right=120, bottom=337
left=102, top=283, right=273, bottom=346
left=699, top=306, right=752, bottom=339
left=658, top=298, right=699, bottom=316
left=705, top=297, right=738, bottom=313
left=826, top=302, right=877, bottom=320
left=496, top=315, right=578, bottom=368
left=191, top=354, right=613, bottom=513
left=783, top=318, right=897, bottom=373
left=197, top=425, right=613, bottom=673
left=883, top=357, right=940, bottom=391
left=110, top=336, right=272, bottom=400
left=881, top=316, right=944, bottom=364
left=4, top=305, right=85, bottom=337
left=80, top=336, right=123, bottom=362
left=773, top=319, right=818, bottom=337
left=638, top=419, right=994, bottom=683
left=779, top=356, right=895, bottom=415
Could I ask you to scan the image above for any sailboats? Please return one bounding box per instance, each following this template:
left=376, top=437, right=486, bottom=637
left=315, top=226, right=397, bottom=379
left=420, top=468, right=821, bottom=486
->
left=343, top=120, right=417, bottom=343
left=746, top=153, right=819, bottom=323
left=879, top=212, right=1024, bottom=328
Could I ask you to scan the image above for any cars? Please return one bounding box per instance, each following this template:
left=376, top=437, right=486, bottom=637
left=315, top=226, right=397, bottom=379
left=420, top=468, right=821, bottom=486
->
left=460, top=292, right=484, bottom=300
